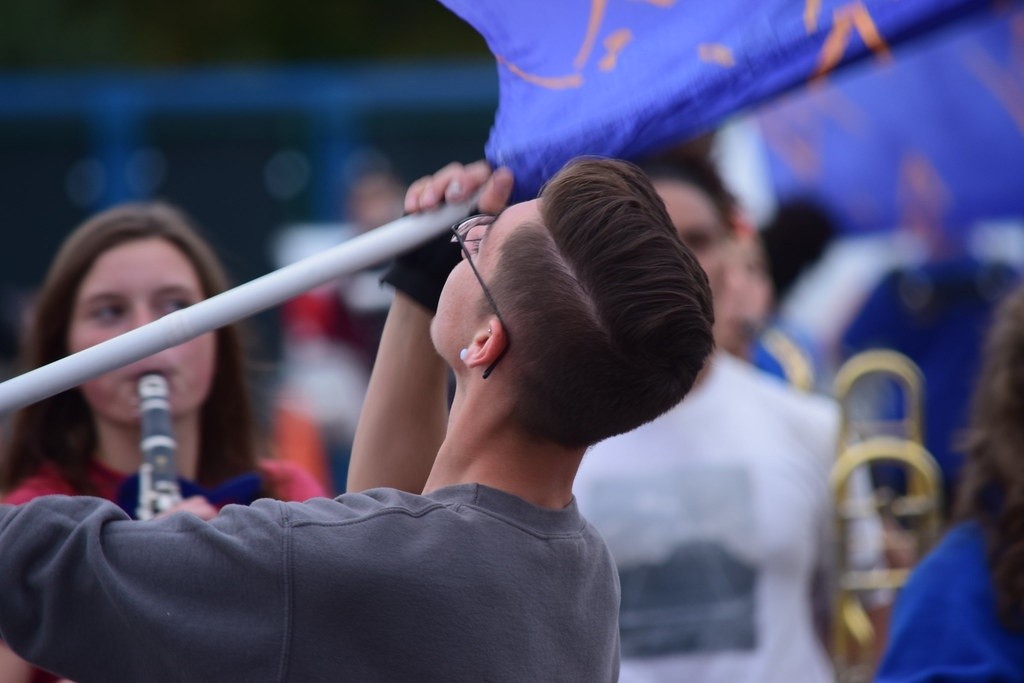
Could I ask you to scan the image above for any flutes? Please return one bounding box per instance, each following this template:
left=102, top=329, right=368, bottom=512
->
left=133, top=375, right=184, bottom=521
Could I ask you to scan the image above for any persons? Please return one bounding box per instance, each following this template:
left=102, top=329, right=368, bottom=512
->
left=872, top=284, right=1024, bottom=683
left=0, top=203, right=327, bottom=682
left=572, top=144, right=852, bottom=683
left=0, top=154, right=717, bottom=682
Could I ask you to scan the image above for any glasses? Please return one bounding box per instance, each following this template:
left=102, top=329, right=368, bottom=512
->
left=448, top=214, right=511, bottom=379
